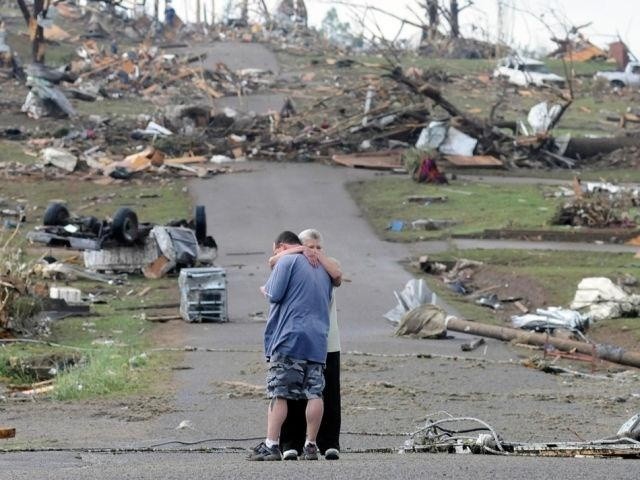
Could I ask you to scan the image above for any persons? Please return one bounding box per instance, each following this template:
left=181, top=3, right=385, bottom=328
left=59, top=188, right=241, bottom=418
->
left=241, top=231, right=333, bottom=461
left=267, top=229, right=343, bottom=460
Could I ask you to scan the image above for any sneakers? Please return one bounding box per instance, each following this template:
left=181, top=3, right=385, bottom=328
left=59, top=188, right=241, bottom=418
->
left=248, top=442, right=281, bottom=461
left=301, top=443, right=319, bottom=461
left=281, top=443, right=298, bottom=460
left=326, top=447, right=339, bottom=459
